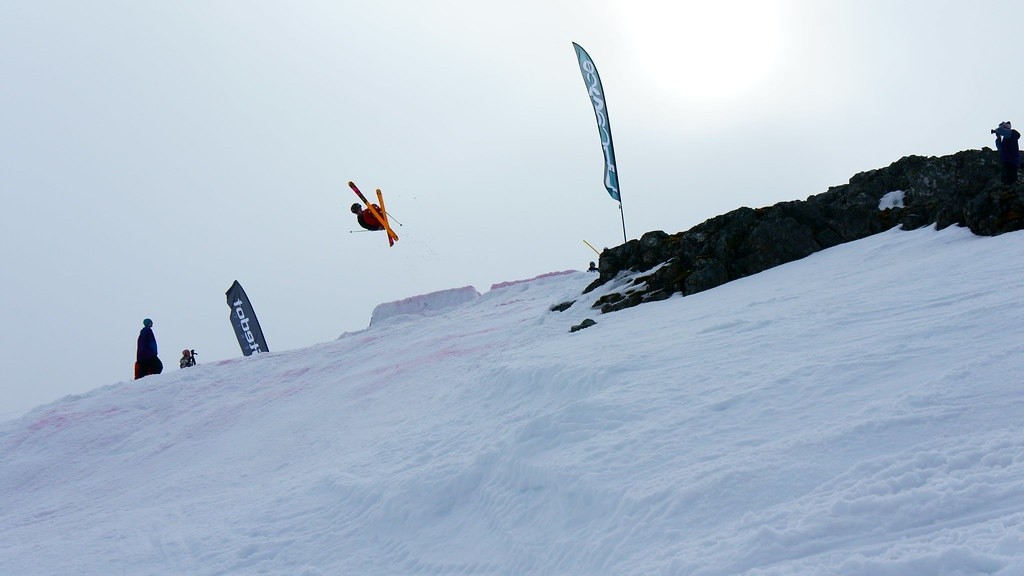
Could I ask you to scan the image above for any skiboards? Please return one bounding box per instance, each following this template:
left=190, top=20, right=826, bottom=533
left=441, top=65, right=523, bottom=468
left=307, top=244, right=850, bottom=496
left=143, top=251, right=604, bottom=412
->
left=347, top=181, right=398, bottom=247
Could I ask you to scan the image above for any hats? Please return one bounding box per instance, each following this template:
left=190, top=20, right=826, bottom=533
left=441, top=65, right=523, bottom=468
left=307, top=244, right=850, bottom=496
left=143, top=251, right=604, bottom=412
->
left=143, top=318, right=151, bottom=325
left=351, top=203, right=361, bottom=213
left=182, top=350, right=189, bottom=355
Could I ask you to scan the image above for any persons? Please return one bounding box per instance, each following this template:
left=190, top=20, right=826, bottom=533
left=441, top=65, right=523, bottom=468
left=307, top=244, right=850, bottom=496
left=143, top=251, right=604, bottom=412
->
left=587, top=262, right=599, bottom=272
left=351, top=203, right=386, bottom=231
left=137, top=318, right=163, bottom=378
left=996, top=122, right=1020, bottom=186
left=180, top=350, right=195, bottom=368
